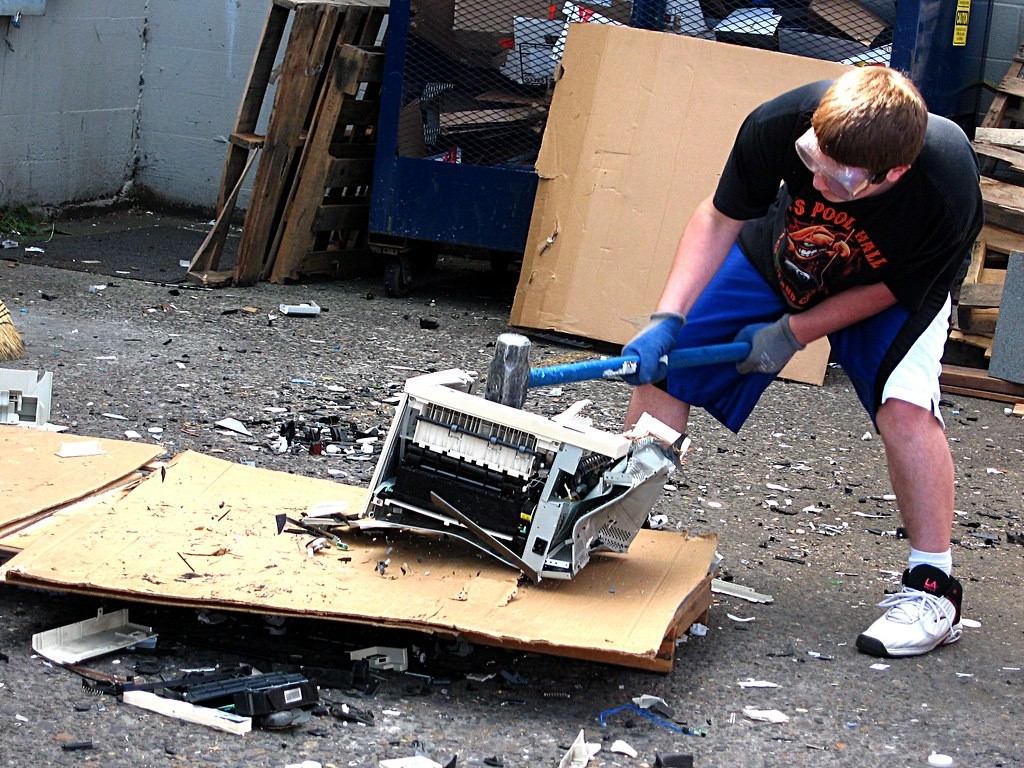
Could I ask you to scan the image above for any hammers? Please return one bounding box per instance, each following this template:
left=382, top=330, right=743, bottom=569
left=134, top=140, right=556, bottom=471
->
left=483, top=332, right=751, bottom=411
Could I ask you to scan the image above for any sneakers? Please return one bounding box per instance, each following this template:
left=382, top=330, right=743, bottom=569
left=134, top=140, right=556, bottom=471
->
left=855, top=563, right=964, bottom=659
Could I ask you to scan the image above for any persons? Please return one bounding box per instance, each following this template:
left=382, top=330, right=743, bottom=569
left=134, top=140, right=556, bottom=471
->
left=623, top=66, right=985, bottom=658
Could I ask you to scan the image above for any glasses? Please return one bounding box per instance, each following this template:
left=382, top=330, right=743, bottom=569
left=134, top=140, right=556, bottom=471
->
left=793, top=126, right=876, bottom=201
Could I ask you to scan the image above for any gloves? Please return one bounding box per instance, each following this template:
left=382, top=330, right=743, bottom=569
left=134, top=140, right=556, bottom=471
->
left=733, top=312, right=807, bottom=374
left=620, top=313, right=685, bottom=385
